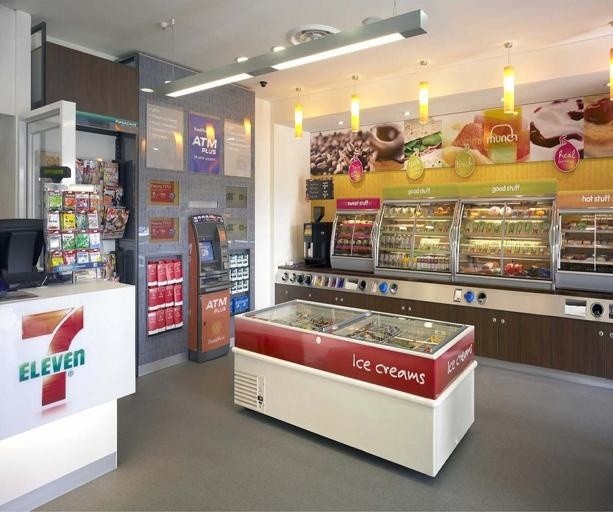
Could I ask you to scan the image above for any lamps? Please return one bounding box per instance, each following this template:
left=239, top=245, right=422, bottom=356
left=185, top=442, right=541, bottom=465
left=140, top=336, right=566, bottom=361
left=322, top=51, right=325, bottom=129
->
left=162, top=1, right=428, bottom=98
left=351, top=74, right=359, bottom=132
left=501, top=44, right=515, bottom=114
left=418, top=61, right=429, bottom=125
left=294, top=92, right=303, bottom=139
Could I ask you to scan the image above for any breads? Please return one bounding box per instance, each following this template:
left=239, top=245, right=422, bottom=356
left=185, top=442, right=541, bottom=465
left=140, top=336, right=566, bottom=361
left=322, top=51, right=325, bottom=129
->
left=584, top=98, right=613, bottom=158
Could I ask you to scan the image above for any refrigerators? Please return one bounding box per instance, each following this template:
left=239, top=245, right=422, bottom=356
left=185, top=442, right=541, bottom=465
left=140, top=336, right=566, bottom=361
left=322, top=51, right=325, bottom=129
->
left=331, top=179, right=613, bottom=294
left=229, top=300, right=477, bottom=479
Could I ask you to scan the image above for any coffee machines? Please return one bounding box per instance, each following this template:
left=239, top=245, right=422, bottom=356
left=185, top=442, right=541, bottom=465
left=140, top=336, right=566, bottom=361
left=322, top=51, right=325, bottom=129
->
left=303, top=206, right=332, bottom=267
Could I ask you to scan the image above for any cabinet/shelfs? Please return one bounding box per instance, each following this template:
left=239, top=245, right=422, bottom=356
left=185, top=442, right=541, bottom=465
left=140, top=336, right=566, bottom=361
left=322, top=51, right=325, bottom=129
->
left=274, top=285, right=612, bottom=382
left=329, top=198, right=613, bottom=290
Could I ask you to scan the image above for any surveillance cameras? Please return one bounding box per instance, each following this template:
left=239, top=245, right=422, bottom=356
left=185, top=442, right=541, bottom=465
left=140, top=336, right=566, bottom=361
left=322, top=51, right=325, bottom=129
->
left=259, top=79, right=269, bottom=87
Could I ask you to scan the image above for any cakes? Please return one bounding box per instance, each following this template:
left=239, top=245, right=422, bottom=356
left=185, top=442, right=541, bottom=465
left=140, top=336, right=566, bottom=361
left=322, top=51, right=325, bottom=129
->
left=530, top=96, right=584, bottom=148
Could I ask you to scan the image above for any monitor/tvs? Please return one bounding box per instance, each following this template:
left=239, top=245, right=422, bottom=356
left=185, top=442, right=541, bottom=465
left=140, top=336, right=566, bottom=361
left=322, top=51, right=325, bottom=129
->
left=0, top=220, right=46, bottom=287
